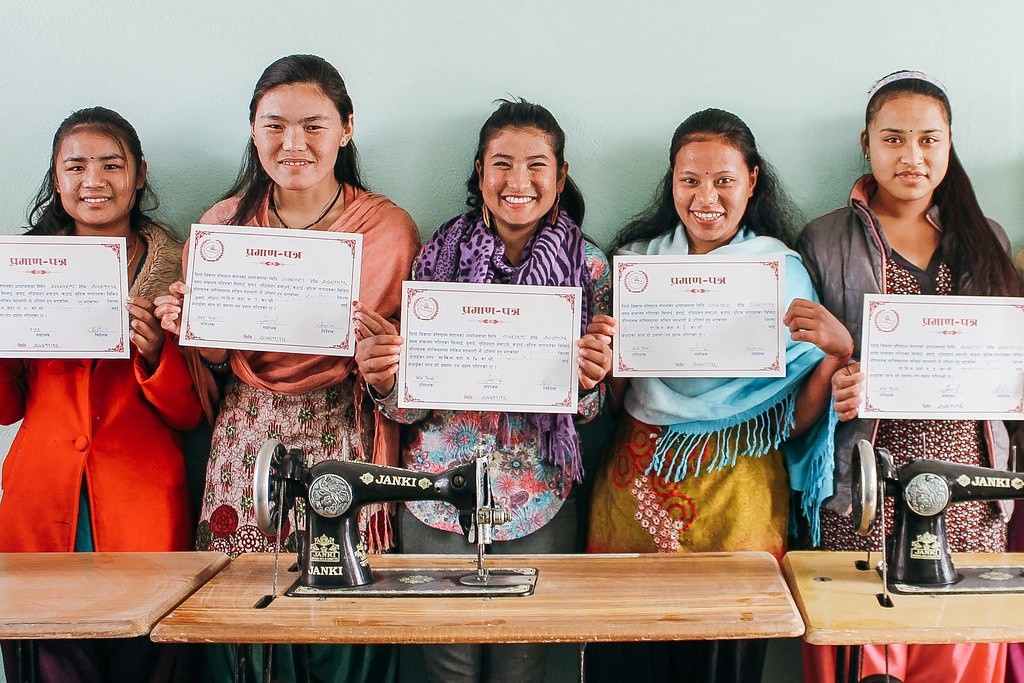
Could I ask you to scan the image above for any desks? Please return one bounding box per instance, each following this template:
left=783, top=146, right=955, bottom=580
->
left=0, top=548, right=235, bottom=683
left=781, top=551, right=1024, bottom=683
left=148, top=551, right=806, bottom=683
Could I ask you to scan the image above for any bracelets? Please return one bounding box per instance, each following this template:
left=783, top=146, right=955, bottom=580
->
left=202, top=357, right=230, bottom=368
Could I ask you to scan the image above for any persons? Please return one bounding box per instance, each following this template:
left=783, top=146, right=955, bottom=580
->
left=791, top=71, right=1024, bottom=683
left=353, top=95, right=619, bottom=683
left=0, top=107, right=204, bottom=683
left=155, top=54, right=419, bottom=683
left=586, top=109, right=855, bottom=683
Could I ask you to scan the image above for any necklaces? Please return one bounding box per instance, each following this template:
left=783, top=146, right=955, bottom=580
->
left=272, top=179, right=342, bottom=229
left=128, top=234, right=139, bottom=267
